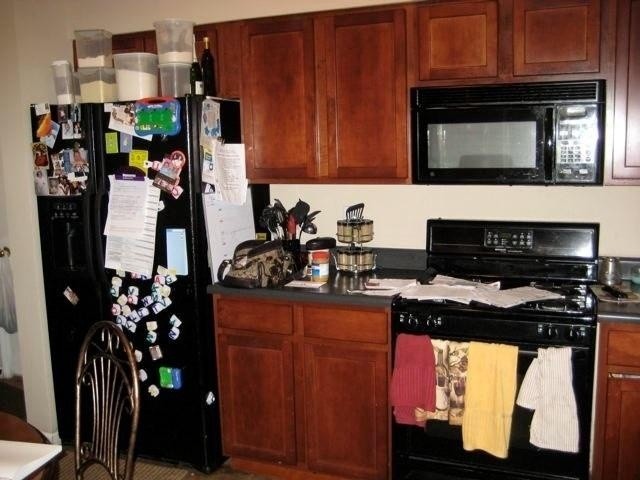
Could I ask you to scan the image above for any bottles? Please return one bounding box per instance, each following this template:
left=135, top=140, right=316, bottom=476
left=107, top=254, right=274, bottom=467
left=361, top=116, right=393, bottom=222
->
left=203, top=36, right=218, bottom=94
left=191, top=59, right=203, bottom=96
left=335, top=249, right=375, bottom=273
left=337, top=218, right=374, bottom=244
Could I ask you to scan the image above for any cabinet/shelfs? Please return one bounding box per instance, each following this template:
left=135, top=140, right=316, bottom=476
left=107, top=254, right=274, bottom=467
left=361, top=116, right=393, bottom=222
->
left=603, top=1, right=640, bottom=186
left=214, top=285, right=392, bottom=479
left=408, top=0, right=614, bottom=86
left=593, top=323, right=640, bottom=479
left=217, top=1, right=404, bottom=185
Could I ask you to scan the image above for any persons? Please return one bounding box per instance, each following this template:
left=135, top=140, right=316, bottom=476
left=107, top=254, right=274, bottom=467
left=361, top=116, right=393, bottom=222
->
left=64, top=124, right=72, bottom=137
left=49, top=178, right=58, bottom=196
left=73, top=123, right=81, bottom=134
left=59, top=177, right=70, bottom=196
left=71, top=179, right=78, bottom=195
left=34, top=171, right=48, bottom=196
left=74, top=105, right=79, bottom=122
left=70, top=140, right=86, bottom=176
left=62, top=147, right=71, bottom=174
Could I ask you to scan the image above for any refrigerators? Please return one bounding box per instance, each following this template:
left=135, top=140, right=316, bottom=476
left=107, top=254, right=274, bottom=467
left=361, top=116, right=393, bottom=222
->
left=30, top=95, right=246, bottom=473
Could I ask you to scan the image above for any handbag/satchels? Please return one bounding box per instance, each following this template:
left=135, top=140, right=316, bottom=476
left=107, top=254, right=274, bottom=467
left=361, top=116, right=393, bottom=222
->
left=217, top=237, right=297, bottom=289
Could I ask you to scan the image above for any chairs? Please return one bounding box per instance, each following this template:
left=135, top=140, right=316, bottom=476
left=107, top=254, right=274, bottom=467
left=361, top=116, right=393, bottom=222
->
left=49, top=320, right=141, bottom=479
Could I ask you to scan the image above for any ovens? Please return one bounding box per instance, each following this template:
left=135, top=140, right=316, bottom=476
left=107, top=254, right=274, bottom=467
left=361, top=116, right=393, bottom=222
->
left=391, top=308, right=597, bottom=479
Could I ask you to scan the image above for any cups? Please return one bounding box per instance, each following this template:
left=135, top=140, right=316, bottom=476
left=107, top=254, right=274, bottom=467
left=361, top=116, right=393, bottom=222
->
left=307, top=238, right=336, bottom=281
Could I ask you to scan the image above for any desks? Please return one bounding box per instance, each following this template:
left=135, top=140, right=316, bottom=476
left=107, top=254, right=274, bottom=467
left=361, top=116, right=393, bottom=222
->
left=0, top=410, right=62, bottom=480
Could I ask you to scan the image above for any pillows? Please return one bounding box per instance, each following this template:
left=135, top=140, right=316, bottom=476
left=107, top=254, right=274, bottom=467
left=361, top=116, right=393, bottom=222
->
left=464, top=341, right=519, bottom=462
left=518, top=344, right=581, bottom=460
left=449, top=341, right=467, bottom=434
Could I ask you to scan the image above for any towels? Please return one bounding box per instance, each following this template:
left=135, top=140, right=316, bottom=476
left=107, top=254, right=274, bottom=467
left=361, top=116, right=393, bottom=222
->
left=388, top=332, right=438, bottom=431
left=414, top=339, right=448, bottom=424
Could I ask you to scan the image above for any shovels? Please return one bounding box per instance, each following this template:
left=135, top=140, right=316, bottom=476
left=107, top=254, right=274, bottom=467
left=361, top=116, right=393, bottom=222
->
left=346, top=203, right=365, bottom=249
left=275, top=208, right=291, bottom=241
left=295, top=201, right=310, bottom=223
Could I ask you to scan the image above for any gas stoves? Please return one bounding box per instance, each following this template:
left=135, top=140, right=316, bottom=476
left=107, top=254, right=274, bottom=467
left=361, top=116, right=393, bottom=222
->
left=395, top=268, right=601, bottom=323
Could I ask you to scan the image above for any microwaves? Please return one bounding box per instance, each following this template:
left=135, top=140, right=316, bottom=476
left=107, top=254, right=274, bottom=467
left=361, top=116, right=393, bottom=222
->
left=410, top=81, right=605, bottom=185
